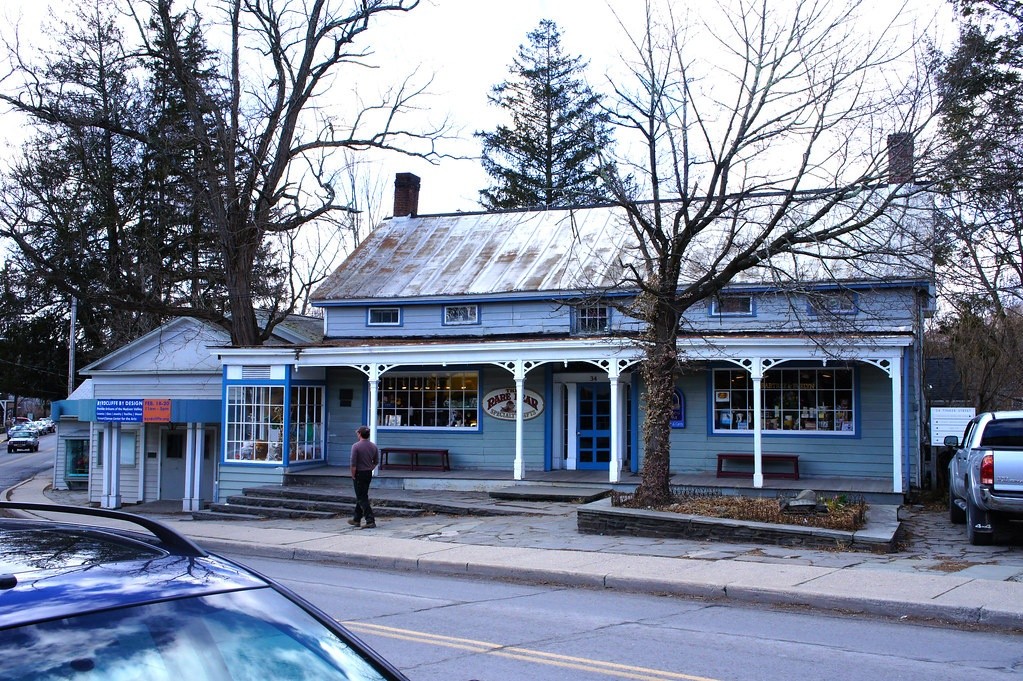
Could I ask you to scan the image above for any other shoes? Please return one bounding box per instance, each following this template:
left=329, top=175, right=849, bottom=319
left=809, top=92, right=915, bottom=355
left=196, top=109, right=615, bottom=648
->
left=362, top=523, right=376, bottom=528
left=348, top=520, right=359, bottom=526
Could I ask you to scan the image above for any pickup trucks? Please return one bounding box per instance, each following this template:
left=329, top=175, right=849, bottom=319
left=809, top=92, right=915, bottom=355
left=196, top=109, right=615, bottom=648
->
left=944, top=409, right=1023, bottom=547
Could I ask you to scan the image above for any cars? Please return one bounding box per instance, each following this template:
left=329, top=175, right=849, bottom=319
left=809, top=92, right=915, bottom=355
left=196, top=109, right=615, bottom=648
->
left=7, top=417, right=56, bottom=441
left=6, top=430, right=39, bottom=453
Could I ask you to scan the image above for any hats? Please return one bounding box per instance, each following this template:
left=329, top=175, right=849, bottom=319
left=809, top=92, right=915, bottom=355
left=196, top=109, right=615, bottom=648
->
left=358, top=426, right=370, bottom=434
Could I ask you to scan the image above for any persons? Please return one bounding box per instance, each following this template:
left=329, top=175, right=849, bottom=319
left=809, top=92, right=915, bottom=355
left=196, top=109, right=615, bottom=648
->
left=348, top=427, right=379, bottom=528
left=5, top=418, right=13, bottom=439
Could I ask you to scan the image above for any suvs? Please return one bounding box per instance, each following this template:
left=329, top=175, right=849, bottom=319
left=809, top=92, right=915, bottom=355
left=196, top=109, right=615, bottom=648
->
left=0, top=496, right=409, bottom=681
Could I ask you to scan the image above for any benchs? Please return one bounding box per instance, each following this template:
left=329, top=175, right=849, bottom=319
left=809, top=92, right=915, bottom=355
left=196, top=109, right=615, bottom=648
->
left=379, top=448, right=451, bottom=472
left=717, top=453, right=800, bottom=481
